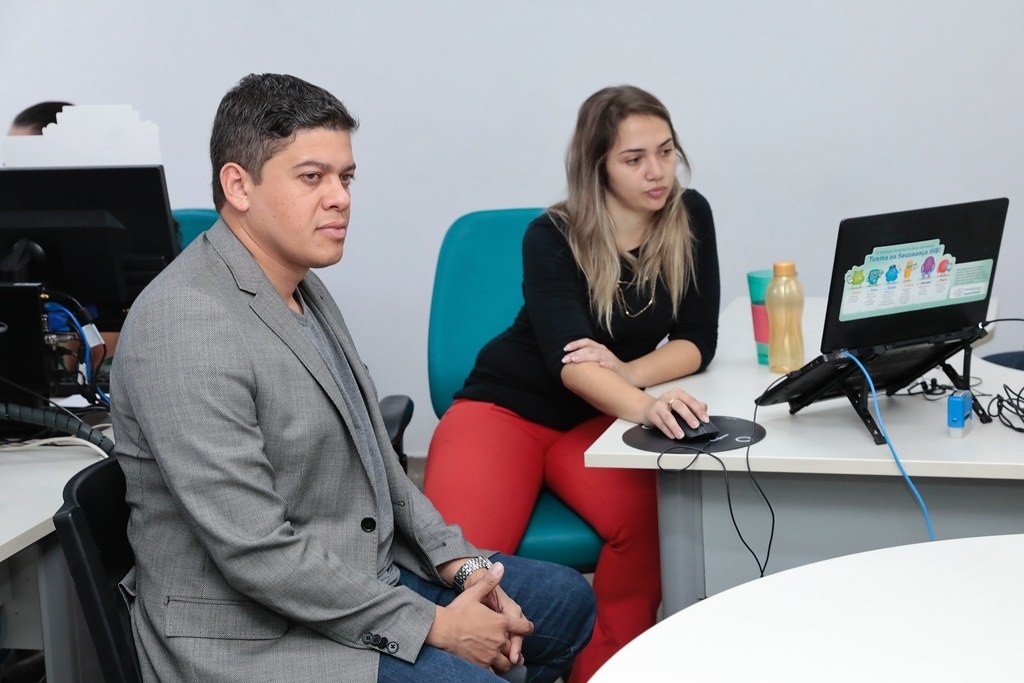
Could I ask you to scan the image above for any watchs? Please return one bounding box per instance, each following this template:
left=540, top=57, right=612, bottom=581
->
left=453, top=556, right=492, bottom=591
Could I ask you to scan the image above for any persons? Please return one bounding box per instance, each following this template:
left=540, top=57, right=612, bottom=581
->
left=109, top=73, right=597, bottom=683
left=420, top=86, right=720, bottom=682
left=8, top=100, right=182, bottom=369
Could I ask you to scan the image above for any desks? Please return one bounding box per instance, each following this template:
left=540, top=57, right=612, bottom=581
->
left=580, top=293, right=1024, bottom=620
left=587, top=529, right=1024, bottom=683
left=0, top=427, right=114, bottom=683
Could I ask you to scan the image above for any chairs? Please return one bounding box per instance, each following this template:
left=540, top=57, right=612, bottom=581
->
left=375, top=205, right=602, bottom=573
left=49, top=454, right=143, bottom=683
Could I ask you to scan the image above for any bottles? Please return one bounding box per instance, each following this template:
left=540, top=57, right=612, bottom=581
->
left=765, top=260, right=804, bottom=373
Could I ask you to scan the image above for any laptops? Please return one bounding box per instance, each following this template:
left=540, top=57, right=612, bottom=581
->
left=756, top=197, right=1010, bottom=407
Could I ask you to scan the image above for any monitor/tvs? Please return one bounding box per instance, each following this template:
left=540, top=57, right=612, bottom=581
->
left=0, top=164, right=179, bottom=332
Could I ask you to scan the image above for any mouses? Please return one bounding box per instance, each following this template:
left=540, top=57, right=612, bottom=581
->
left=670, top=409, right=719, bottom=443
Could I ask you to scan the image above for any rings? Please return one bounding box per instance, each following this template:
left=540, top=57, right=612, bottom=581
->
left=668, top=398, right=674, bottom=409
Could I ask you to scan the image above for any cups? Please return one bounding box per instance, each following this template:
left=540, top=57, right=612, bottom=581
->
left=747, top=270, right=775, bottom=365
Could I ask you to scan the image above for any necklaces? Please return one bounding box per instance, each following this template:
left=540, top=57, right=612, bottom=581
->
left=618, top=280, right=656, bottom=317
